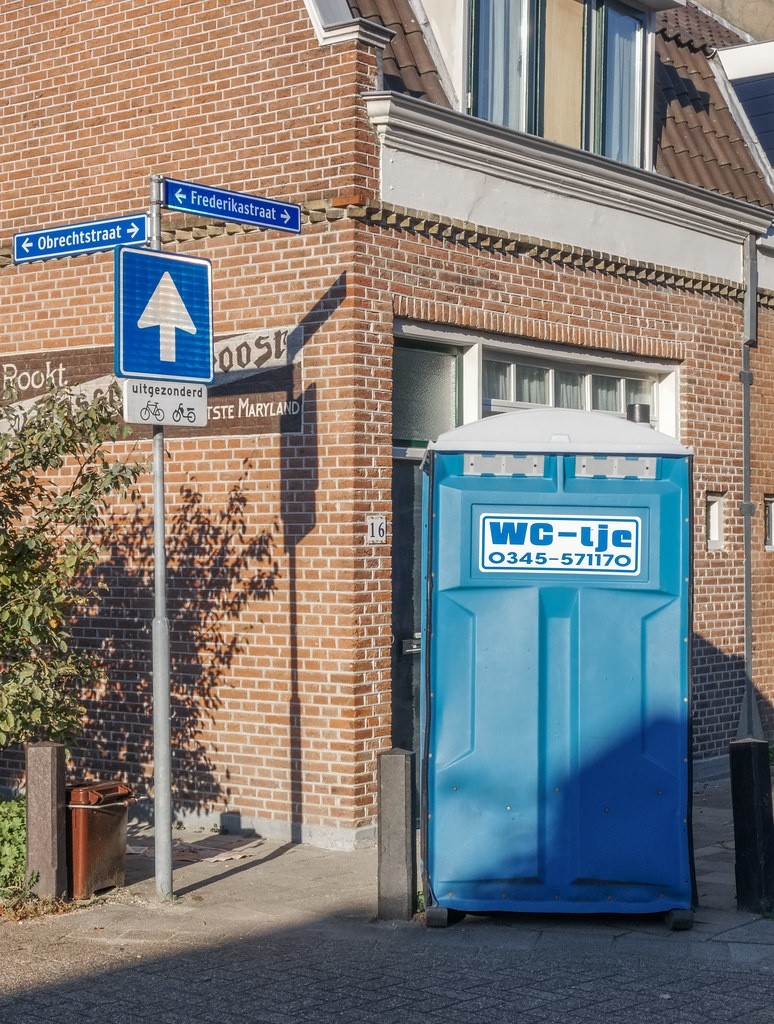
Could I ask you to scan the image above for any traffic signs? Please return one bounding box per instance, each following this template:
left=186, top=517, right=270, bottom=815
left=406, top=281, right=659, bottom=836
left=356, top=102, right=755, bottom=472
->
left=162, top=177, right=302, bottom=235
left=11, top=212, right=148, bottom=264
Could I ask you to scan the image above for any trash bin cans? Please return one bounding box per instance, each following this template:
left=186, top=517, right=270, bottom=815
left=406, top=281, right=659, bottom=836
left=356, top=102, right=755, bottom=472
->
left=65, top=782, right=147, bottom=898
